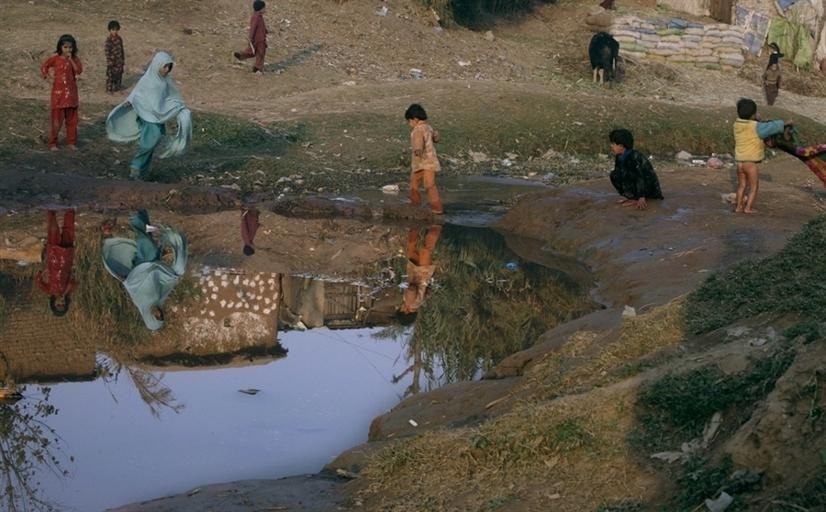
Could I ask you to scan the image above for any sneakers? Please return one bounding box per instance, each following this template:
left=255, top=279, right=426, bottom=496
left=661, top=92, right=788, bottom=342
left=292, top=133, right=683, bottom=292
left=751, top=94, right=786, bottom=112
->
left=234, top=52, right=264, bottom=75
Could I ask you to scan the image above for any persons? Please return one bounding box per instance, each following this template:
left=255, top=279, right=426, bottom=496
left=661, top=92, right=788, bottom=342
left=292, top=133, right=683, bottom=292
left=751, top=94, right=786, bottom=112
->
left=96, top=208, right=188, bottom=332
left=606, top=125, right=665, bottom=211
left=239, top=205, right=262, bottom=260
left=762, top=62, right=783, bottom=106
left=727, top=95, right=795, bottom=215
left=231, top=0, right=268, bottom=75
left=765, top=41, right=787, bottom=71
left=33, top=206, right=80, bottom=319
left=104, top=50, right=194, bottom=180
left=394, top=221, right=443, bottom=328
left=103, top=17, right=125, bottom=98
left=39, top=30, right=83, bottom=154
left=401, top=100, right=444, bottom=218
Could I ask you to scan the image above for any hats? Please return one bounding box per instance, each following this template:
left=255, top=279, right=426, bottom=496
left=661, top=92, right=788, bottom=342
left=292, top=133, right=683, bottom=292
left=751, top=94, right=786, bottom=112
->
left=767, top=43, right=778, bottom=51
